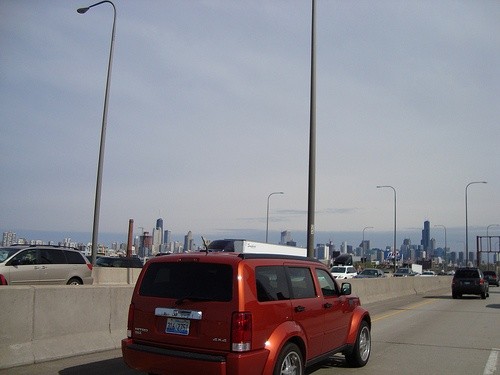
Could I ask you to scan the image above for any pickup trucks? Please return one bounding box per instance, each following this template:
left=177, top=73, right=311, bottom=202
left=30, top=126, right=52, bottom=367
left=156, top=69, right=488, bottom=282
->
left=391, top=268, right=417, bottom=277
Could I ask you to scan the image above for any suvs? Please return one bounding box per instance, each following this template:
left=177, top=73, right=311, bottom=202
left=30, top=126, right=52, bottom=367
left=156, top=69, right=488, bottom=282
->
left=120, top=238, right=373, bottom=374
left=451, top=267, right=490, bottom=299
left=96, top=255, right=144, bottom=268
left=483, top=270, right=499, bottom=287
left=0, top=243, right=94, bottom=285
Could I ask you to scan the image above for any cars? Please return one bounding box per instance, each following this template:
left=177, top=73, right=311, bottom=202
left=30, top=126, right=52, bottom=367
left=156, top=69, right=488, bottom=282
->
left=420, top=271, right=437, bottom=276
left=352, top=268, right=384, bottom=279
left=329, top=265, right=358, bottom=279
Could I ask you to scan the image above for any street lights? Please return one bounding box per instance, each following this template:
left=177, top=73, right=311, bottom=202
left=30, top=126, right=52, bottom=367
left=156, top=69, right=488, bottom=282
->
left=77, top=0, right=118, bottom=267
left=362, top=226, right=375, bottom=263
left=465, top=181, right=489, bottom=267
left=434, top=224, right=447, bottom=260
left=375, top=185, right=396, bottom=273
left=265, top=191, right=285, bottom=243
